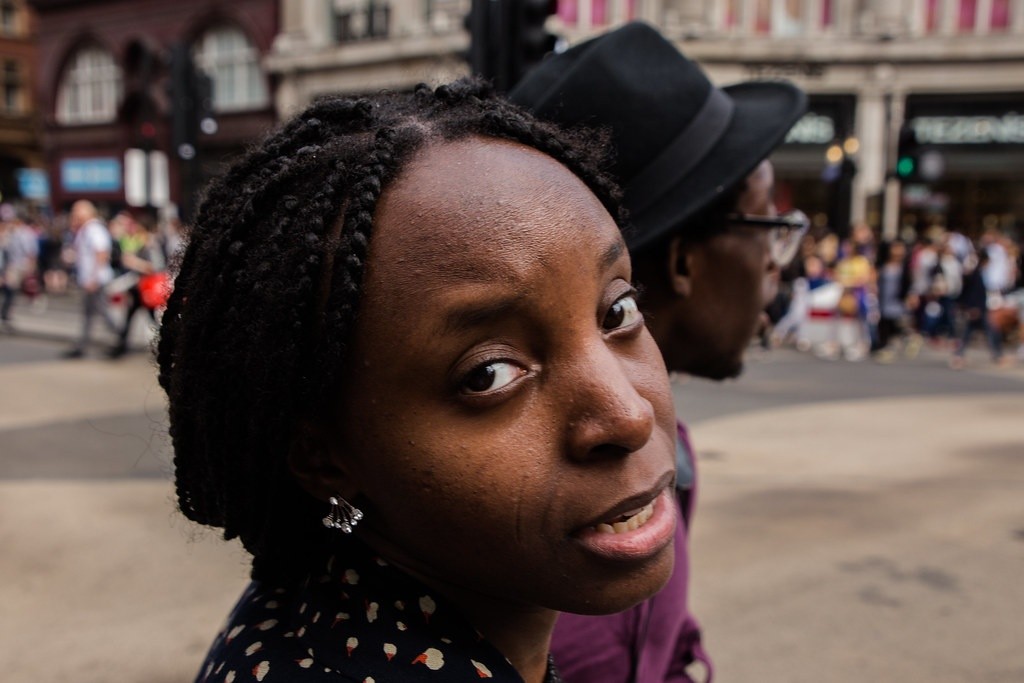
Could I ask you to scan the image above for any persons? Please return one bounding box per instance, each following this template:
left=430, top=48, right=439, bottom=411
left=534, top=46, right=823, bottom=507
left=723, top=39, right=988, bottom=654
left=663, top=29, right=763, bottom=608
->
left=0, top=199, right=184, bottom=361
left=754, top=215, right=1024, bottom=368
left=159, top=75, right=674, bottom=683
left=506, top=20, right=807, bottom=683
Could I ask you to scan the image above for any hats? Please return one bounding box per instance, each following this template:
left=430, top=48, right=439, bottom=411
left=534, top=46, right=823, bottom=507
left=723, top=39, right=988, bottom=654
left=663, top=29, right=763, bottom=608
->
left=480, top=20, right=809, bottom=257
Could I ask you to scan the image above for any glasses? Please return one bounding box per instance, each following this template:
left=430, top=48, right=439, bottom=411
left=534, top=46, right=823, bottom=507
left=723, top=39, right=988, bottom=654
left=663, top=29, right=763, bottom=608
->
left=728, top=199, right=809, bottom=269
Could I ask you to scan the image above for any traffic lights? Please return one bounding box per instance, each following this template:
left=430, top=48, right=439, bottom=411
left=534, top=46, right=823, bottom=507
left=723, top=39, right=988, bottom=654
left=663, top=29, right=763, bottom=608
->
left=500, top=1, right=564, bottom=88
left=109, top=33, right=182, bottom=160
left=897, top=125, right=921, bottom=185
left=456, top=0, right=512, bottom=87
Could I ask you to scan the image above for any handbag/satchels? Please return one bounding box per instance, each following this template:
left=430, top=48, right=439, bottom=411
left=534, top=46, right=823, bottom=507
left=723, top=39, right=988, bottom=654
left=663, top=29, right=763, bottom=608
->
left=139, top=271, right=175, bottom=306
left=22, top=277, right=42, bottom=295
